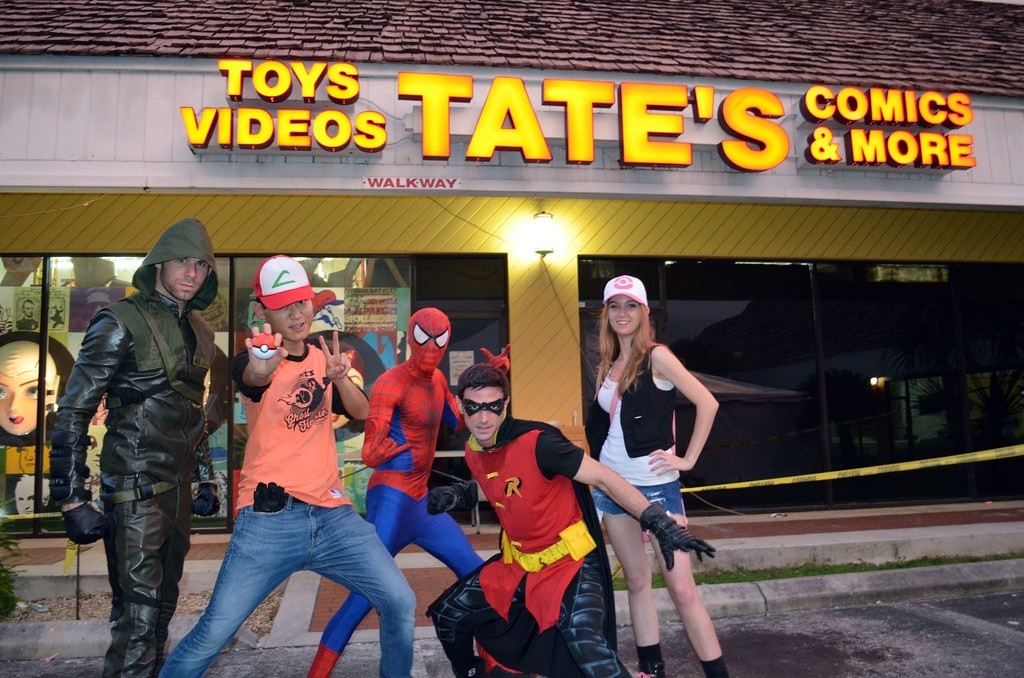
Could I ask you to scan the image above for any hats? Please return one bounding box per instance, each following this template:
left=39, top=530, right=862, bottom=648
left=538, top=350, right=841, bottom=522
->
left=253, top=255, right=315, bottom=311
left=603, top=275, right=648, bottom=307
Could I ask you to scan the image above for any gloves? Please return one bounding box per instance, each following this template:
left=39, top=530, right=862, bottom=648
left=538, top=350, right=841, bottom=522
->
left=253, top=481, right=290, bottom=513
left=63, top=503, right=106, bottom=545
left=192, top=482, right=220, bottom=516
left=639, top=504, right=716, bottom=571
left=426, top=486, right=458, bottom=516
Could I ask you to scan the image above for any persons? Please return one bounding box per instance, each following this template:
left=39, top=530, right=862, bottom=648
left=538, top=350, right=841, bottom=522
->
left=51, top=219, right=218, bottom=678
left=585, top=276, right=728, bottom=678
left=309, top=308, right=511, bottom=678
left=428, top=363, right=716, bottom=678
left=155, top=255, right=417, bottom=678
